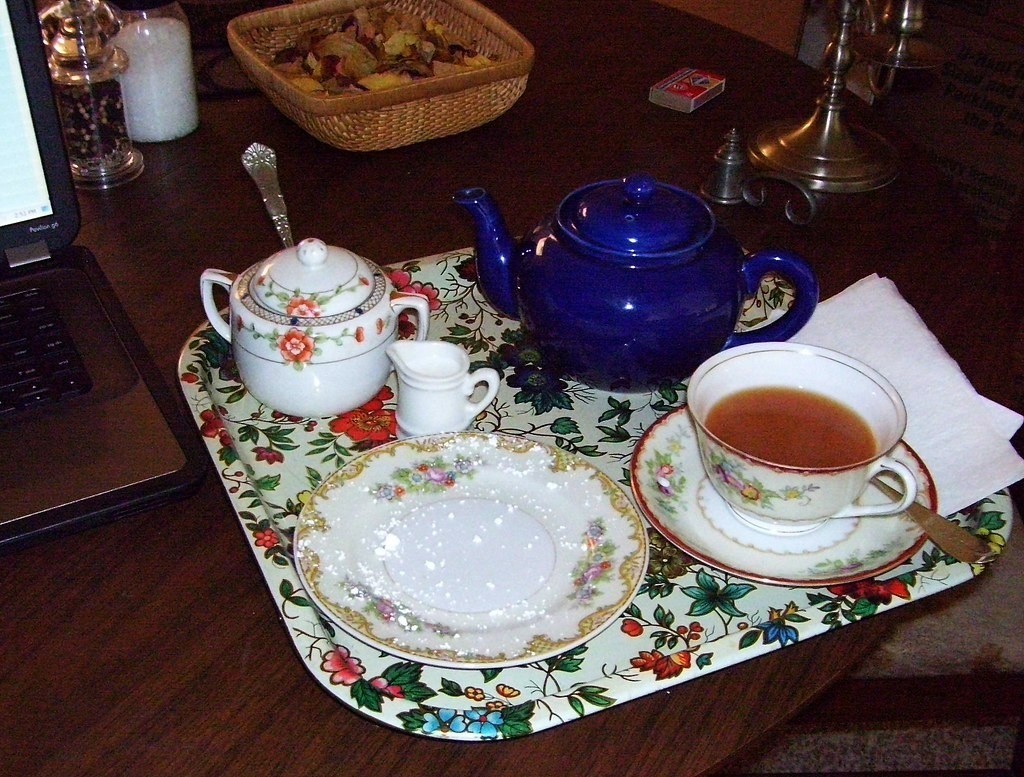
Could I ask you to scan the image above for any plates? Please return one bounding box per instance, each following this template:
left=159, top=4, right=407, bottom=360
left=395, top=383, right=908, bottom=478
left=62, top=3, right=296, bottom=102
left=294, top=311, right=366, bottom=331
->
left=293, top=428, right=649, bottom=671
left=629, top=401, right=940, bottom=587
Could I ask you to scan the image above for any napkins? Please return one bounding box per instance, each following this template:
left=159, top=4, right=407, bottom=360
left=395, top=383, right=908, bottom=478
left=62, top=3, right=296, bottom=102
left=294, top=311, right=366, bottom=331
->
left=761, top=271, right=1024, bottom=518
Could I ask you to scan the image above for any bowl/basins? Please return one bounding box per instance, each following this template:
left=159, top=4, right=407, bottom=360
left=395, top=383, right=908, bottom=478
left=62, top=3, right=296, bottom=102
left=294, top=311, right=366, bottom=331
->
left=198, top=239, right=430, bottom=421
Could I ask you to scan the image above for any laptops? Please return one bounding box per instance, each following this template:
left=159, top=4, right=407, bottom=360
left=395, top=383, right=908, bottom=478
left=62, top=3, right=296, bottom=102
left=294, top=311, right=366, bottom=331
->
left=1, top=0, right=201, bottom=556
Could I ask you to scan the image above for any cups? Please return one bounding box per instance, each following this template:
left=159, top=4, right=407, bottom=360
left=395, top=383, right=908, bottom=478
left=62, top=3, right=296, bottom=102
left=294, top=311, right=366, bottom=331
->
left=687, top=340, right=909, bottom=534
left=384, top=335, right=501, bottom=442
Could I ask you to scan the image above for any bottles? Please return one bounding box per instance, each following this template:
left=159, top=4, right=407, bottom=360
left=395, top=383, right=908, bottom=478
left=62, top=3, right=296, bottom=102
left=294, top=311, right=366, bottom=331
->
left=106, top=0, right=201, bottom=144
left=35, top=0, right=145, bottom=190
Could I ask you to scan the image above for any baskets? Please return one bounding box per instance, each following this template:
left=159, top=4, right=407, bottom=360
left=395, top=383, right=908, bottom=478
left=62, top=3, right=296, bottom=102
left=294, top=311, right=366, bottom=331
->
left=227, top=0, right=536, bottom=155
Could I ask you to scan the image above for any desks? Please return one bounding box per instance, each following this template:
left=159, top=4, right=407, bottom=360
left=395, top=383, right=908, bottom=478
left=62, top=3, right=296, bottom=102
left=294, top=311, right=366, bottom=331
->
left=0, top=0, right=1024, bottom=777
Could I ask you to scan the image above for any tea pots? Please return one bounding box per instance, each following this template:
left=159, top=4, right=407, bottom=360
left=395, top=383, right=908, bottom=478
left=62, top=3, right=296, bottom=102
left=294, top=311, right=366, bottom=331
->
left=452, top=173, right=820, bottom=392
